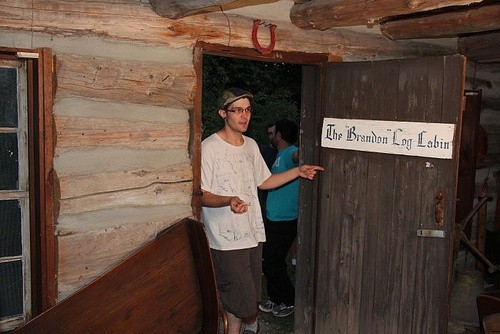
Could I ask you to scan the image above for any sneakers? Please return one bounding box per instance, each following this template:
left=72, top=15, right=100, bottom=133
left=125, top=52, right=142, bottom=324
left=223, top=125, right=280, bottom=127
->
left=273, top=303, right=295, bottom=317
left=258, top=300, right=283, bottom=312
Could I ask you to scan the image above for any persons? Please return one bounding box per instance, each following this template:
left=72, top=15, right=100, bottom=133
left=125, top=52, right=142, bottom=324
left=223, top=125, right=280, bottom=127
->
left=259, top=119, right=300, bottom=317
left=201, top=90, right=325, bottom=334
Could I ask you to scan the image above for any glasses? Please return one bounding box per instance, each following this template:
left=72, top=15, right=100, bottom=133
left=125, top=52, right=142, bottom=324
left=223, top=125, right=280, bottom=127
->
left=224, top=109, right=253, bottom=114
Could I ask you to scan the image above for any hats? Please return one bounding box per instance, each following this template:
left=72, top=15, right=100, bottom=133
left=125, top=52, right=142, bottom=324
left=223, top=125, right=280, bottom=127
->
left=217, top=88, right=254, bottom=109
